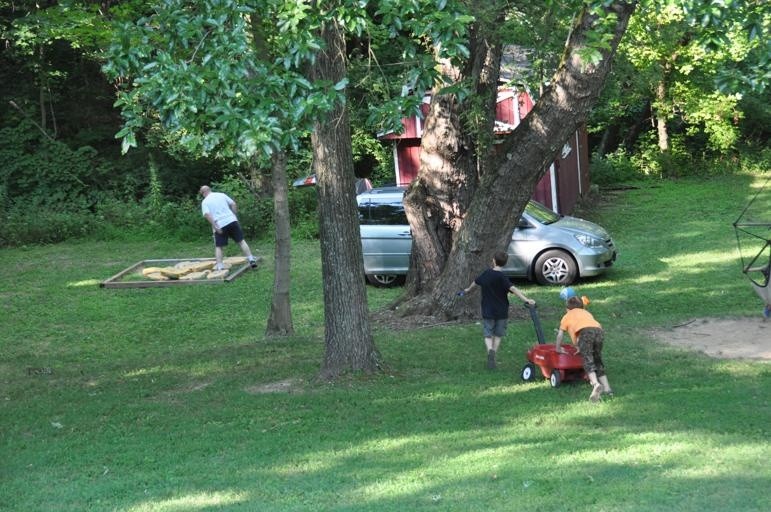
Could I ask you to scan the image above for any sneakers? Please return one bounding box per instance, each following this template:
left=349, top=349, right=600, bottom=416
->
left=488, top=349, right=495, bottom=368
left=250, top=261, right=257, bottom=268
left=589, top=383, right=612, bottom=404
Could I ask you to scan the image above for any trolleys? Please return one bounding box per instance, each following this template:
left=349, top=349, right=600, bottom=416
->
left=522, top=298, right=592, bottom=388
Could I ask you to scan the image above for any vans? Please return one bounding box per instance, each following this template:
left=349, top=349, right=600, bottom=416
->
left=355, top=185, right=616, bottom=289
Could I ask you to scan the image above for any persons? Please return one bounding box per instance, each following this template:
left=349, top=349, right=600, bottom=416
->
left=465, top=251, right=536, bottom=369
left=557, top=295, right=615, bottom=402
left=200, top=185, right=258, bottom=274
